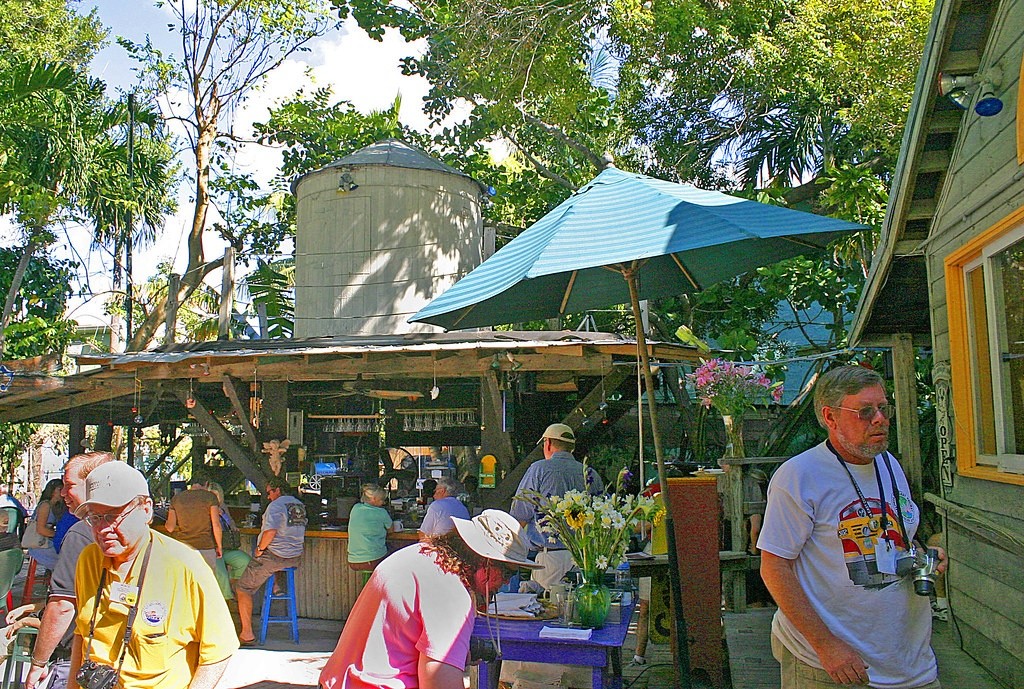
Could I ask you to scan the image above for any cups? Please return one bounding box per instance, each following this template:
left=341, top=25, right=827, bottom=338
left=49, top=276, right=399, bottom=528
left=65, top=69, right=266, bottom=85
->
left=555, top=592, right=576, bottom=624
left=544, top=586, right=566, bottom=604
left=394, top=521, right=404, bottom=532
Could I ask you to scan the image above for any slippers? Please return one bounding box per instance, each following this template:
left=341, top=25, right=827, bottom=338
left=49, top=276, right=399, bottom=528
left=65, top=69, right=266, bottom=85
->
left=238, top=638, right=257, bottom=645
left=275, top=589, right=285, bottom=596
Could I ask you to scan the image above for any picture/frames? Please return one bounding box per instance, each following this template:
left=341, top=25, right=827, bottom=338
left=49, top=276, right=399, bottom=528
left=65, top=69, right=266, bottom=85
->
left=285, top=471, right=302, bottom=487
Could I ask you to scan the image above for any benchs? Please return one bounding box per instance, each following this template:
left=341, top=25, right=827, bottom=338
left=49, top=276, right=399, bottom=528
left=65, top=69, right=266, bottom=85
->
left=713, top=457, right=789, bottom=555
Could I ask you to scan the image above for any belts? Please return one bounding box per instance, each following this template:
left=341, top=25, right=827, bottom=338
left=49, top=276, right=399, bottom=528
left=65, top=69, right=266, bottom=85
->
left=538, top=547, right=568, bottom=551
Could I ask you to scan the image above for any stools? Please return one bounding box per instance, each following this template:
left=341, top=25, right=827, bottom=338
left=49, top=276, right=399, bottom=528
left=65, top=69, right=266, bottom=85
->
left=23, top=551, right=51, bottom=615
left=357, top=571, right=373, bottom=585
left=257, top=566, right=299, bottom=647
left=1, top=626, right=50, bottom=689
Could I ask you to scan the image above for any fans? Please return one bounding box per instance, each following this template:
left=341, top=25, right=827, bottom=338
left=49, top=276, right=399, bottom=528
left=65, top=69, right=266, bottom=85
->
left=363, top=445, right=419, bottom=502
left=292, top=379, right=423, bottom=404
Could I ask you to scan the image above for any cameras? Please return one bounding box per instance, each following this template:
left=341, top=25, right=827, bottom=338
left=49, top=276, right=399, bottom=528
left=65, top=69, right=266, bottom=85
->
left=77, top=661, right=118, bottom=689
left=893, top=549, right=942, bottom=596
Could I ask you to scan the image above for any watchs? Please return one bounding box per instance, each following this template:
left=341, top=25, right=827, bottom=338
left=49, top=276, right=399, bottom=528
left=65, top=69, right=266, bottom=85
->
left=257, top=545, right=263, bottom=552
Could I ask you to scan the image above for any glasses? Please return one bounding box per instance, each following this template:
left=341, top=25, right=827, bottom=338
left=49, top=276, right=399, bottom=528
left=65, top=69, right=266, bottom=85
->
left=496, top=561, right=518, bottom=581
left=266, top=489, right=273, bottom=495
left=829, top=405, right=895, bottom=421
left=86, top=503, right=141, bottom=527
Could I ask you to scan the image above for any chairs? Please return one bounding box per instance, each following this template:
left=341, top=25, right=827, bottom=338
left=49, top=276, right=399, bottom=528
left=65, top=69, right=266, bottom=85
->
left=0, top=547, right=26, bottom=625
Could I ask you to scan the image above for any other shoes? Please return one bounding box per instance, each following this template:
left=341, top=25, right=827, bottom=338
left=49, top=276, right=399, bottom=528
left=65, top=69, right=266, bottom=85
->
left=623, top=658, right=647, bottom=670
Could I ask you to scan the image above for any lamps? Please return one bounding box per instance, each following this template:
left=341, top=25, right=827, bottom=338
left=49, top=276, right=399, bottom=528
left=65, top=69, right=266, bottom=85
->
left=936, top=60, right=1004, bottom=124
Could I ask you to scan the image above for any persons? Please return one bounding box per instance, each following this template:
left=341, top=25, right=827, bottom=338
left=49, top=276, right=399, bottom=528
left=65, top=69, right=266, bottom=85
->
left=23, top=450, right=117, bottom=689
left=207, top=482, right=251, bottom=605
left=347, top=483, right=392, bottom=571
left=236, top=478, right=306, bottom=645
left=0, top=478, right=80, bottom=668
left=319, top=508, right=544, bottom=689
left=508, top=424, right=603, bottom=593
left=754, top=367, right=949, bottom=688
left=462, top=474, right=480, bottom=502
left=419, top=478, right=471, bottom=541
left=164, top=469, right=222, bottom=574
left=66, top=461, right=240, bottom=689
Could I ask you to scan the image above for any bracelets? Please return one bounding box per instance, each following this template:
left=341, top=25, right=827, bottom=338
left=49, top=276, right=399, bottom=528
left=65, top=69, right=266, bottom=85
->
left=30, top=656, right=49, bottom=667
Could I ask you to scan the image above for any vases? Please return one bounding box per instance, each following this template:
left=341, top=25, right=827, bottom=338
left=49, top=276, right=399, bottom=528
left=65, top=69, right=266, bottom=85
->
left=722, top=415, right=748, bottom=458
left=573, top=568, right=617, bottom=630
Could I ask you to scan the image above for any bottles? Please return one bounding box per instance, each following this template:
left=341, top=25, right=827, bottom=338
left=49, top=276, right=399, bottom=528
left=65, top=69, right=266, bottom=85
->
left=615, top=561, right=632, bottom=606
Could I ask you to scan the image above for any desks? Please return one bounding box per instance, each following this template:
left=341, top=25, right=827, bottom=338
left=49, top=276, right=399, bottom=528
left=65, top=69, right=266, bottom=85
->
left=465, top=589, right=640, bottom=689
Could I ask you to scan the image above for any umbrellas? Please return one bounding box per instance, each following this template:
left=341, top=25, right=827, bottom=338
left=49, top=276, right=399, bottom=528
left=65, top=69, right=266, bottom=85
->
left=403, top=162, right=875, bottom=688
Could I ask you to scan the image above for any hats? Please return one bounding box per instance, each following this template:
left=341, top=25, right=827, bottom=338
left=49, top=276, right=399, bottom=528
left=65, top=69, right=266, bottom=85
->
left=0, top=511, right=10, bottom=527
left=450, top=509, right=545, bottom=570
left=537, top=423, right=576, bottom=445
left=74, top=461, right=148, bottom=518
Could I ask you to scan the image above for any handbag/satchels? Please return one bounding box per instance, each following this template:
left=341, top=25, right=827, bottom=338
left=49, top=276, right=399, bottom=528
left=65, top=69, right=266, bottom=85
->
left=21, top=519, right=52, bottom=548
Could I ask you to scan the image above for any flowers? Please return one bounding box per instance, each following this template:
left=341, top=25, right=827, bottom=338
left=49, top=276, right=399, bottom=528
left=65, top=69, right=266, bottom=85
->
left=672, top=356, right=787, bottom=416
left=511, top=458, right=673, bottom=570
